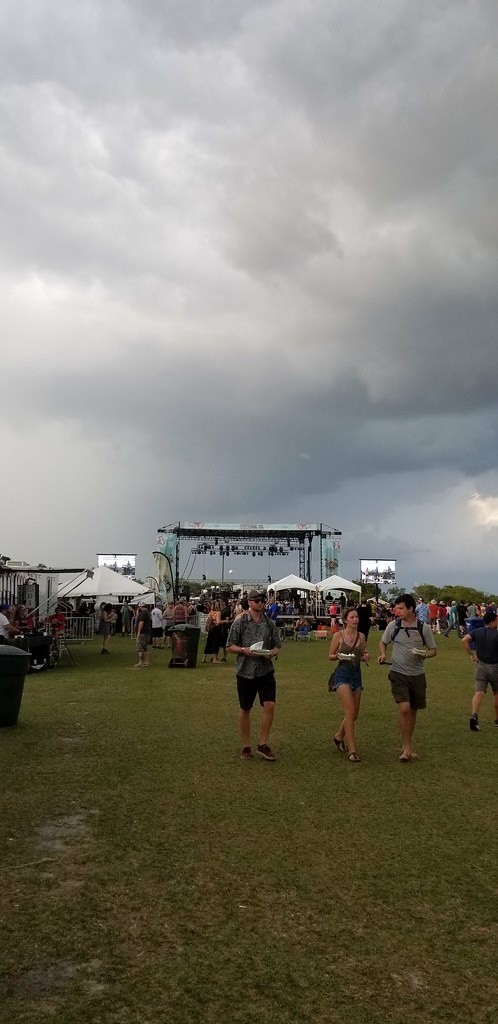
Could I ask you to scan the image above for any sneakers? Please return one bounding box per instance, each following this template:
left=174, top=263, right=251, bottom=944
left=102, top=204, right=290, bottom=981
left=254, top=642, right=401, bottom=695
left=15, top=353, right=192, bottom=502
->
left=469, top=713, right=480, bottom=731
left=152, top=646, right=162, bottom=650
left=241, top=744, right=252, bottom=760
left=256, top=744, right=276, bottom=760
left=494, top=720, right=498, bottom=729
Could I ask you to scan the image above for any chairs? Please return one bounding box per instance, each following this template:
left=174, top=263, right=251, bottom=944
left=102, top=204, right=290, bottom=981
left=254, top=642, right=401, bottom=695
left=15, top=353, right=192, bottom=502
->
left=278, top=623, right=328, bottom=641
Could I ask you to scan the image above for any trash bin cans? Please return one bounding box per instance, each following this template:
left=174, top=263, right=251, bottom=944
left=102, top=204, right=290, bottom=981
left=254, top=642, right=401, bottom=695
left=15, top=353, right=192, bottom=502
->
left=0, top=645, right=32, bottom=728
left=168, top=624, right=201, bottom=668
left=464, top=617, right=484, bottom=650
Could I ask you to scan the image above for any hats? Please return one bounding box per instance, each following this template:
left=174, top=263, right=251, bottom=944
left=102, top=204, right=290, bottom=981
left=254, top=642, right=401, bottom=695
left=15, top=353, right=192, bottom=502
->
left=138, top=601, right=145, bottom=607
left=156, top=602, right=162, bottom=605
left=249, top=591, right=267, bottom=599
left=431, top=599, right=437, bottom=605
left=452, top=601, right=456, bottom=604
left=17, top=605, right=28, bottom=612
left=1, top=604, right=10, bottom=609
left=440, top=601, right=445, bottom=605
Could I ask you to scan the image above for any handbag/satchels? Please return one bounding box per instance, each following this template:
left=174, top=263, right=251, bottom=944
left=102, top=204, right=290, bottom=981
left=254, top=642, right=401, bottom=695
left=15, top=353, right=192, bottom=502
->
left=328, top=673, right=335, bottom=692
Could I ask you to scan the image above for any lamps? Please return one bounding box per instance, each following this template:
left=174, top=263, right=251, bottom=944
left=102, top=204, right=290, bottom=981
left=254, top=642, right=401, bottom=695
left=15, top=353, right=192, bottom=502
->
left=189, top=537, right=295, bottom=558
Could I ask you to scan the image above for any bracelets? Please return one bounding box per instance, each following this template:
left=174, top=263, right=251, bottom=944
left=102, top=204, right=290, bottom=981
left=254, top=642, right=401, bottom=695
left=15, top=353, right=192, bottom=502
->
left=241, top=647, right=244, bottom=653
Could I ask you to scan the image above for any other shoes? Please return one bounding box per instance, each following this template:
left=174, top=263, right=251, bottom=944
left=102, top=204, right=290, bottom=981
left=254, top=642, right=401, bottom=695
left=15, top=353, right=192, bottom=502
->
left=202, top=660, right=210, bottom=663
left=213, top=661, right=221, bottom=664
left=210, top=657, right=219, bottom=663
left=221, top=657, right=226, bottom=662
left=101, top=648, right=111, bottom=655
left=134, top=662, right=151, bottom=668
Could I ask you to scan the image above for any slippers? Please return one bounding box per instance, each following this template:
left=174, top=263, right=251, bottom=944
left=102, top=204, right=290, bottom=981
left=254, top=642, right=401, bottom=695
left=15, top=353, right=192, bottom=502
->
left=347, top=747, right=361, bottom=762
left=399, top=745, right=419, bottom=761
left=333, top=734, right=347, bottom=754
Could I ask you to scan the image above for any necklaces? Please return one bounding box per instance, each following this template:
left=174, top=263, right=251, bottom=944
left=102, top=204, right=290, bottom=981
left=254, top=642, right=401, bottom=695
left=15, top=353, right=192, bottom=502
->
left=346, top=630, right=354, bottom=642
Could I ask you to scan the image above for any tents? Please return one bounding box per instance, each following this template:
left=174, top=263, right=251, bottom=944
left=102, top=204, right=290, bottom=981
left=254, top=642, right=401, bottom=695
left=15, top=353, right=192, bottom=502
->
left=57, top=565, right=152, bottom=597
left=267, top=574, right=362, bottom=614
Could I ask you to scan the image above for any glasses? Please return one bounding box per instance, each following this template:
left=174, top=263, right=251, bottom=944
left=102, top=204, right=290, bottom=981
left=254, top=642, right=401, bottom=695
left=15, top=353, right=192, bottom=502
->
left=250, top=599, right=265, bottom=604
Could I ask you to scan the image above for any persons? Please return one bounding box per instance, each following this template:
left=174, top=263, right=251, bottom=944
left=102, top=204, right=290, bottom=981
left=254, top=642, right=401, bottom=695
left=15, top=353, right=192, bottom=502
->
left=462, top=612, right=498, bottom=731
left=0, top=603, right=35, bottom=645
left=226, top=589, right=282, bottom=759
left=57, top=588, right=498, bottom=669
left=49, top=608, right=65, bottom=636
left=377, top=594, right=437, bottom=760
left=327, top=606, right=369, bottom=761
